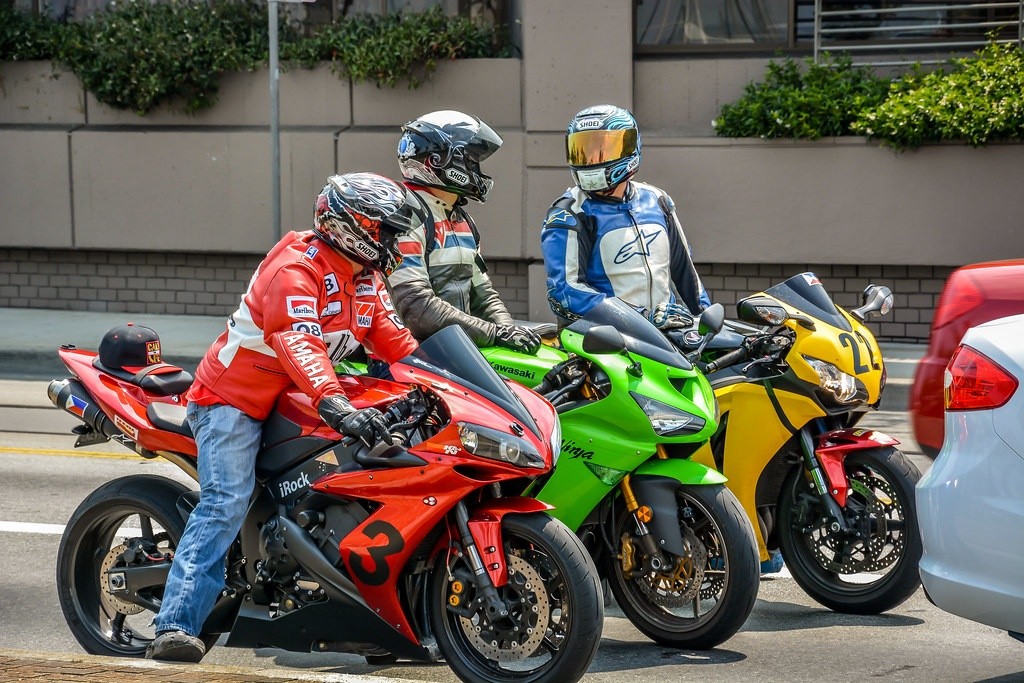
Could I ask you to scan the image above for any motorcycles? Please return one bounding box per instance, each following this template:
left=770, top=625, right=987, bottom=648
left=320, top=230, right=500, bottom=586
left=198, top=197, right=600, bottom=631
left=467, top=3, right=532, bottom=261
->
left=331, top=296, right=761, bottom=649
left=537, top=271, right=925, bottom=614
left=49, top=325, right=604, bottom=683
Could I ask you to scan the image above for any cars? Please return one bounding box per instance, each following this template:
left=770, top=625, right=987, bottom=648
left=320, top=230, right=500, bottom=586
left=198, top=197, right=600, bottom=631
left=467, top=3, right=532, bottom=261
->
left=910, top=258, right=1023, bottom=458
left=915, top=312, right=1023, bottom=646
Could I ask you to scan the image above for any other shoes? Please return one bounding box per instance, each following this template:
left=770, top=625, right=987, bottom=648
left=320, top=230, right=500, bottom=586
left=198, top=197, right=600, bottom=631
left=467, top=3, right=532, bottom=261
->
left=145, top=631, right=206, bottom=663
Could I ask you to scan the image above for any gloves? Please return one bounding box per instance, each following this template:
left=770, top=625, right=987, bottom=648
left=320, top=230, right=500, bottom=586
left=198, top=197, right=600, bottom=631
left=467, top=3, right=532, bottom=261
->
left=318, top=395, right=394, bottom=451
left=647, top=302, right=694, bottom=330
left=494, top=324, right=541, bottom=355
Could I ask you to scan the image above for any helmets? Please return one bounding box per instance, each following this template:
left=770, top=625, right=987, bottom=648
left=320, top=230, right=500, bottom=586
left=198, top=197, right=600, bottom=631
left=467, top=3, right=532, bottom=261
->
left=565, top=104, right=642, bottom=192
left=313, top=172, right=429, bottom=279
left=397, top=110, right=504, bottom=204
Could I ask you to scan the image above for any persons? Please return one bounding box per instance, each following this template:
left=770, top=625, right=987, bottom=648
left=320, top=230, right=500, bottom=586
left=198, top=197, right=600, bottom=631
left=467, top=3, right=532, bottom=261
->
left=381, top=107, right=544, bottom=362
left=540, top=100, right=712, bottom=326
left=141, top=170, right=423, bottom=662
